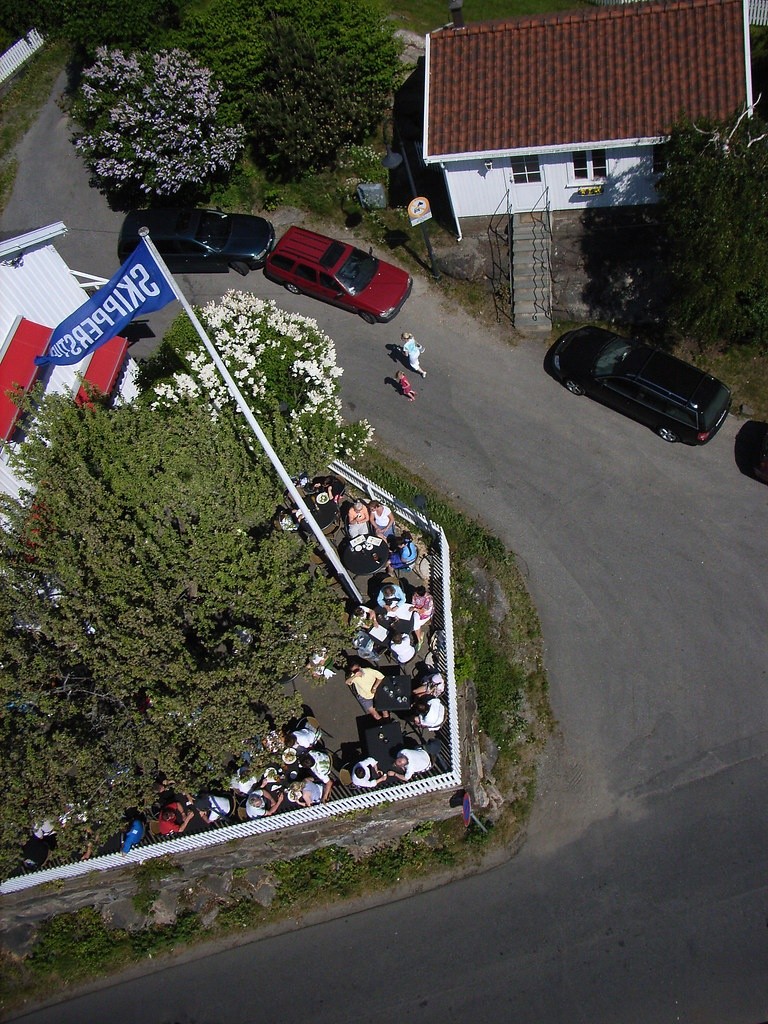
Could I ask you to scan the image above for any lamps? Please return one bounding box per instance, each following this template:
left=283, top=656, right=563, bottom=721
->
left=485, top=159, right=493, bottom=171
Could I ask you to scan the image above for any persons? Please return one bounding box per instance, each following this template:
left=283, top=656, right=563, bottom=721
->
left=186, top=793, right=196, bottom=805
left=348, top=499, right=369, bottom=538
left=119, top=818, right=143, bottom=856
left=150, top=802, right=194, bottom=835
left=350, top=605, right=378, bottom=629
left=377, top=585, right=406, bottom=612
left=368, top=500, right=394, bottom=538
left=237, top=633, right=447, bottom=819
left=397, top=333, right=427, bottom=378
left=278, top=509, right=305, bottom=531
left=393, top=371, right=417, bottom=401
left=291, top=473, right=345, bottom=500
left=196, top=795, right=230, bottom=823
left=409, top=585, right=434, bottom=651
left=386, top=537, right=417, bottom=577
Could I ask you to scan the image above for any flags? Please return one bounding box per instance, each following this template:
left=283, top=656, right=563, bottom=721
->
left=34, top=240, right=176, bottom=366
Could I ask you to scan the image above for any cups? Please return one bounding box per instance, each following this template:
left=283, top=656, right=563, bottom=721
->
left=373, top=553, right=378, bottom=560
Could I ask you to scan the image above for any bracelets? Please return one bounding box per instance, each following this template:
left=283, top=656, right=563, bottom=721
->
left=399, top=349, right=400, bottom=350
left=181, top=811, right=184, bottom=814
left=373, top=688, right=376, bottom=690
left=394, top=772, right=396, bottom=776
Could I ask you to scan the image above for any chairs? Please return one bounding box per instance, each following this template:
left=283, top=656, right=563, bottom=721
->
left=67, top=471, right=448, bottom=863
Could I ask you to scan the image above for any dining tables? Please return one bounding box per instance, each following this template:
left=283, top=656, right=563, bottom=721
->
left=364, top=722, right=408, bottom=785
left=375, top=675, right=412, bottom=711
left=177, top=795, right=210, bottom=831
left=342, top=533, right=388, bottom=576
left=368, top=602, right=413, bottom=648
left=298, top=496, right=339, bottom=533
left=254, top=747, right=306, bottom=811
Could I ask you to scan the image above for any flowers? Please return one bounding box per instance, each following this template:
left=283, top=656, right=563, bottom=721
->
left=578, top=185, right=605, bottom=195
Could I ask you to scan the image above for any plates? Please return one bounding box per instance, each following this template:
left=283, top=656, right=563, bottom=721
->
left=282, top=748, right=297, bottom=764
left=355, top=545, right=362, bottom=551
left=316, top=492, right=329, bottom=504
left=296, top=509, right=304, bottom=519
left=290, top=771, right=297, bottom=779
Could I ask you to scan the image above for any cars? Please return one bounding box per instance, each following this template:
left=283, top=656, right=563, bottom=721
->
left=118, top=205, right=277, bottom=276
left=263, top=225, right=414, bottom=325
left=544, top=325, right=734, bottom=446
